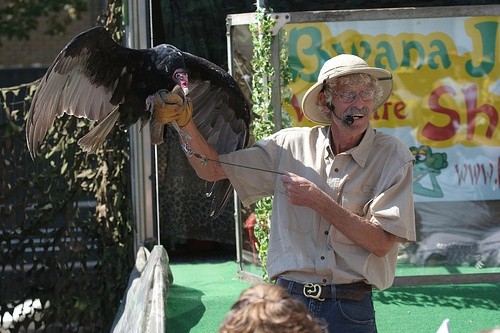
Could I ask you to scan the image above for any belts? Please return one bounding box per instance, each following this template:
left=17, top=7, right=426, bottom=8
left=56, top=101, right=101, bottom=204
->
left=275, top=277, right=373, bottom=302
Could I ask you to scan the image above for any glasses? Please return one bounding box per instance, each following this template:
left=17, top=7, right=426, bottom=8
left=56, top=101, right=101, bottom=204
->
left=330, top=89, right=376, bottom=103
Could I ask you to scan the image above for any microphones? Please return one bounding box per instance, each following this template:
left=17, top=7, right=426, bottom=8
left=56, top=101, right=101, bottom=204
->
left=327, top=102, right=354, bottom=126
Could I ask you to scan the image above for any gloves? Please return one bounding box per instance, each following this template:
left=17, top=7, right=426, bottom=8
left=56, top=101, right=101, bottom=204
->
left=153, top=89, right=193, bottom=127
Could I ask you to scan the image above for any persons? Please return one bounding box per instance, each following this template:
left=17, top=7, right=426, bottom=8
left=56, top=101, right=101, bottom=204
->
left=214, top=282, right=327, bottom=333
left=155, top=52, right=417, bottom=333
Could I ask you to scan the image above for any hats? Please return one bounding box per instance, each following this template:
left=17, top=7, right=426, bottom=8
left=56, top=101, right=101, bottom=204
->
left=301, top=54, right=394, bottom=125
